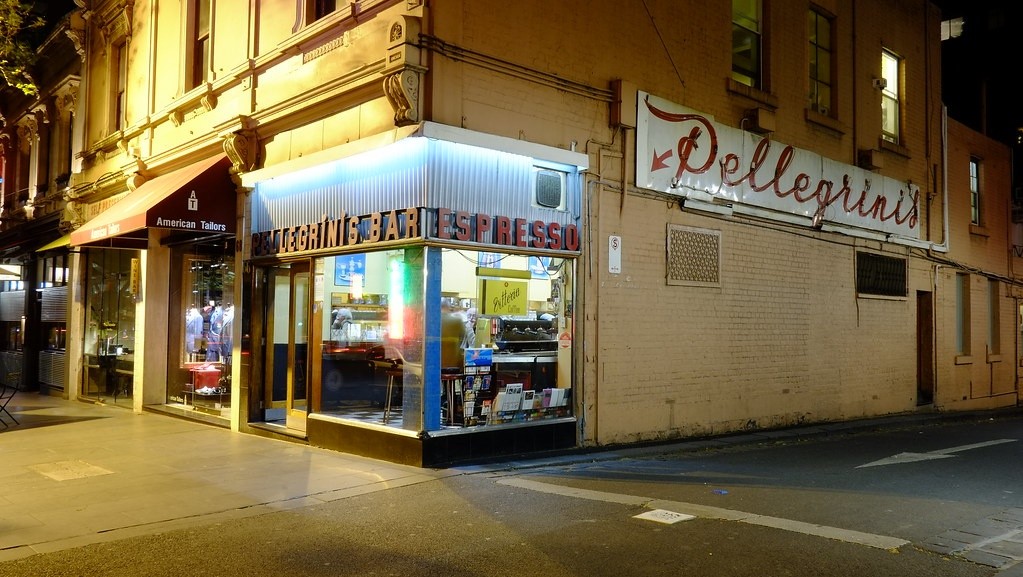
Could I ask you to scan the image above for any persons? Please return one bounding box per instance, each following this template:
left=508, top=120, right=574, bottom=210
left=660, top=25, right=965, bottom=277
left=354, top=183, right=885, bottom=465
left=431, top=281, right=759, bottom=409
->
left=185, top=301, right=234, bottom=363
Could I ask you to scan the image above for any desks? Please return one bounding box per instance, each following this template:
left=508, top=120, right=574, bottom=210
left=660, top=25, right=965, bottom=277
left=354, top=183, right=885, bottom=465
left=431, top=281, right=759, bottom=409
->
left=85, top=353, right=134, bottom=395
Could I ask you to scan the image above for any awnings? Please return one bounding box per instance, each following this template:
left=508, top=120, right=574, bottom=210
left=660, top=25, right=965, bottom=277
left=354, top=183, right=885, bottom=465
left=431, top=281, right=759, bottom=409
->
left=35, top=230, right=75, bottom=259
left=0, top=210, right=62, bottom=241
left=69, top=152, right=239, bottom=251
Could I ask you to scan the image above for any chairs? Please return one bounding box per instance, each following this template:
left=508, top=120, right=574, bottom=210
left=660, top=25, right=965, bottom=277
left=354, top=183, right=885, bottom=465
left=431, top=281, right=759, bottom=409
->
left=0, top=372, right=21, bottom=427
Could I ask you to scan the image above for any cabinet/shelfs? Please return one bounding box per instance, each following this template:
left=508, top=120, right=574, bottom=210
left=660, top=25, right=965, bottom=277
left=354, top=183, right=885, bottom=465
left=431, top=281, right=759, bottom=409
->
left=331, top=292, right=470, bottom=342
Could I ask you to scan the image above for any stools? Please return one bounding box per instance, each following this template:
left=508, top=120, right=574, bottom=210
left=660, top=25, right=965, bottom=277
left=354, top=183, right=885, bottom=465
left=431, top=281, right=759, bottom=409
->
left=442, top=373, right=464, bottom=425
left=383, top=371, right=403, bottom=424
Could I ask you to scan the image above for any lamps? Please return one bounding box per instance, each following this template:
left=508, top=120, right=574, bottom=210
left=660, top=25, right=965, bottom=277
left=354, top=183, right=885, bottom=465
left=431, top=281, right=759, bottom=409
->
left=547, top=258, right=558, bottom=270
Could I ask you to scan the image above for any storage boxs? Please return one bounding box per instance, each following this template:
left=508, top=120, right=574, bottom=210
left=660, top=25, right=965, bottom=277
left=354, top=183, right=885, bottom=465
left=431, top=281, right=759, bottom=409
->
left=528, top=300, right=546, bottom=310
left=459, top=297, right=478, bottom=308
